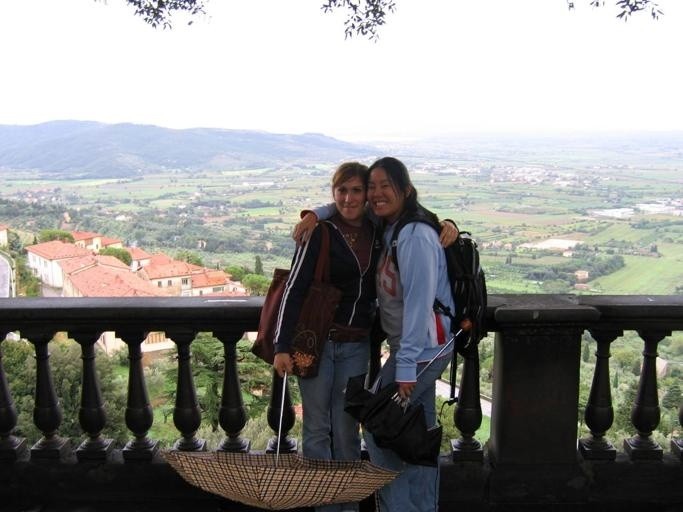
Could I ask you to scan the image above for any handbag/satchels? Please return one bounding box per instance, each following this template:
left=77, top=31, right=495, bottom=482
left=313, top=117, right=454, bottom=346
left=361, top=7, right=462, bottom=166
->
left=250, top=266, right=342, bottom=380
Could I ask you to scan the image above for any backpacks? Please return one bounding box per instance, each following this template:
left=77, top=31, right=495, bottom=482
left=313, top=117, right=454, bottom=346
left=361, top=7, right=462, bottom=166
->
left=390, top=215, right=489, bottom=359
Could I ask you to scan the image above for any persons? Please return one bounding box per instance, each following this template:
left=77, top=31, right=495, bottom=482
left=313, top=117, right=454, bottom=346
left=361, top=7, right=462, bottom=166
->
left=271, top=163, right=378, bottom=511
left=292, top=157, right=456, bottom=512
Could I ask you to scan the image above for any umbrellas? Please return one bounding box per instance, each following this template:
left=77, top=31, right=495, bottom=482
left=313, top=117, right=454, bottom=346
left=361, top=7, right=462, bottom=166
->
left=344, top=318, right=472, bottom=468
left=167, top=373, right=399, bottom=512
